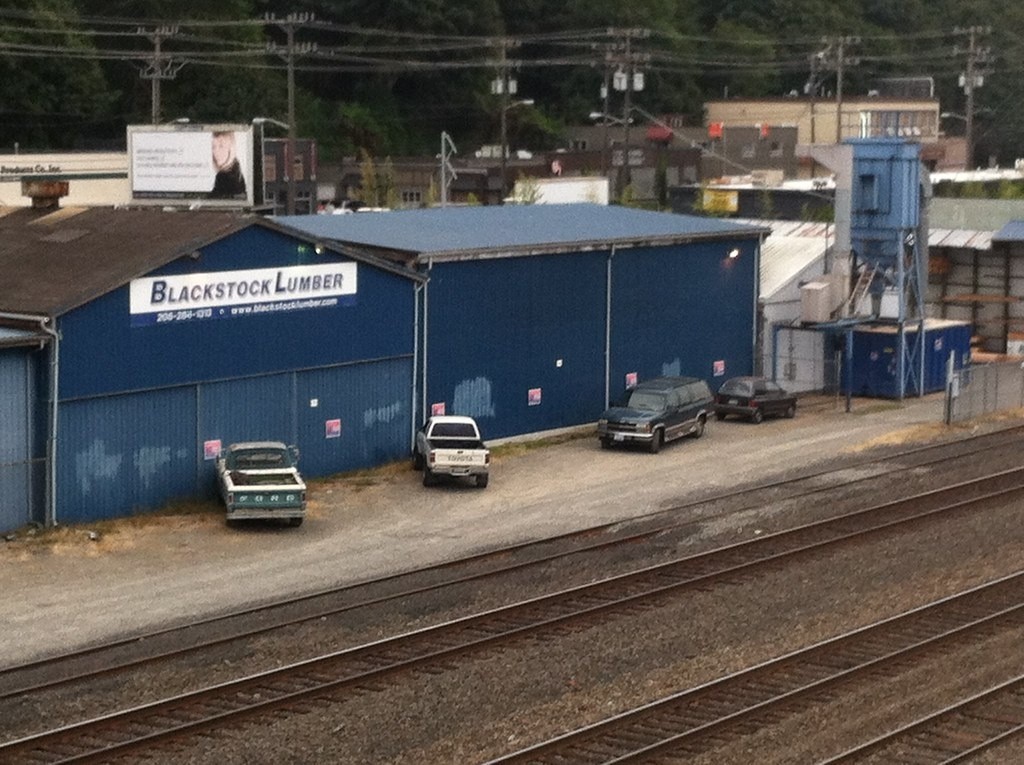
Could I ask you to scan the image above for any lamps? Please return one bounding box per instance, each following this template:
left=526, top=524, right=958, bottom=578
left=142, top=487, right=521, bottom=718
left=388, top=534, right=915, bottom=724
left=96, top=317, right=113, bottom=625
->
left=726, top=247, right=739, bottom=259
left=315, top=243, right=324, bottom=255
left=188, top=250, right=203, bottom=260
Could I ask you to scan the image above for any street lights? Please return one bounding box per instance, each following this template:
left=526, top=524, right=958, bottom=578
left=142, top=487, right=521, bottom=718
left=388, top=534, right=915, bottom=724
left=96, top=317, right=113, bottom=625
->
left=252, top=114, right=296, bottom=217
left=500, top=98, right=535, bottom=205
left=589, top=110, right=630, bottom=203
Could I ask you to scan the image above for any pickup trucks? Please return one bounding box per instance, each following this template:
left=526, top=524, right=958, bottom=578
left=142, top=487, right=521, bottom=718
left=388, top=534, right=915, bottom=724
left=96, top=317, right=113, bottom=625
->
left=413, top=413, right=491, bottom=490
left=215, top=440, right=307, bottom=530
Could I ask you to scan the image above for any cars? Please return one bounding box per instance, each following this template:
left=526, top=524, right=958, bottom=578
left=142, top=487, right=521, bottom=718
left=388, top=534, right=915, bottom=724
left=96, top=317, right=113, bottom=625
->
left=715, top=374, right=799, bottom=425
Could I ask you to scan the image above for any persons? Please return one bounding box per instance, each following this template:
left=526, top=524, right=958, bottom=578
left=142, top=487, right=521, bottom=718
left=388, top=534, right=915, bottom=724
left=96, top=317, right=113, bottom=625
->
left=209, top=131, right=246, bottom=193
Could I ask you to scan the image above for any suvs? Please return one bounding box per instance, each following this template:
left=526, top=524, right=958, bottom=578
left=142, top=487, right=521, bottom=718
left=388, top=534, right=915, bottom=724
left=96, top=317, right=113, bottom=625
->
left=597, top=376, right=719, bottom=454
